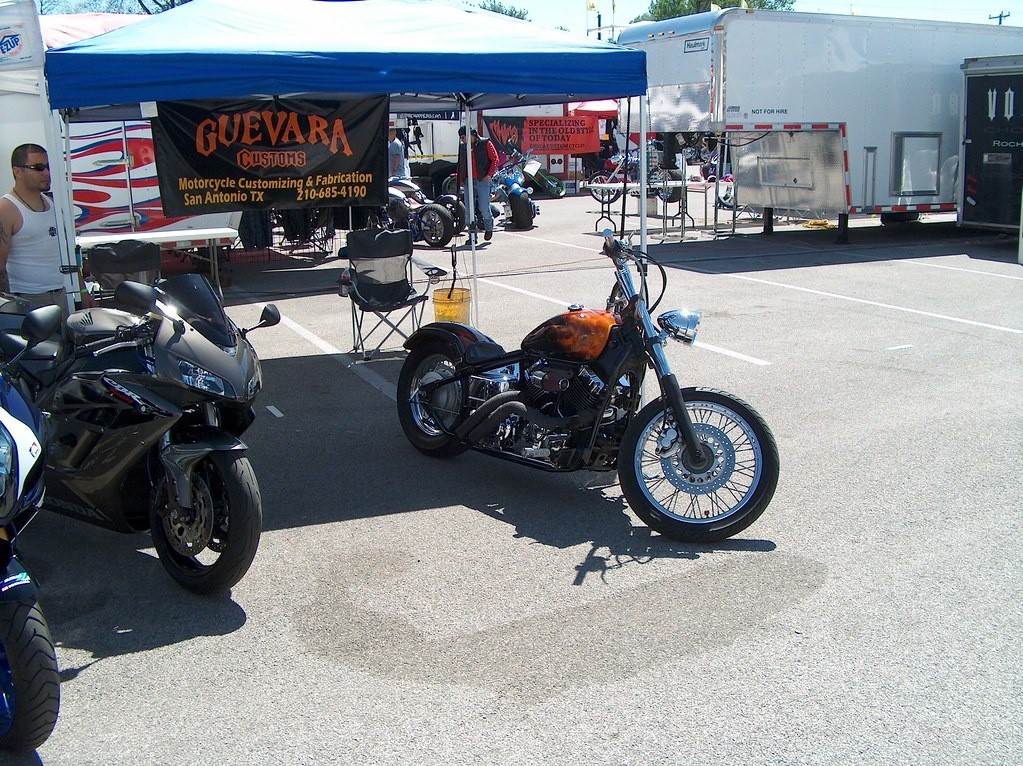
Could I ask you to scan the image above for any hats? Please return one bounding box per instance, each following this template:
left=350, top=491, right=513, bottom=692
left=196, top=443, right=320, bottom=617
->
left=458, top=126, right=472, bottom=136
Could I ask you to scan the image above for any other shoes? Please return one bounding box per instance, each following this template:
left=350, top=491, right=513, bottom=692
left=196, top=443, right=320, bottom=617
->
left=484, top=230, right=492, bottom=240
left=465, top=239, right=478, bottom=245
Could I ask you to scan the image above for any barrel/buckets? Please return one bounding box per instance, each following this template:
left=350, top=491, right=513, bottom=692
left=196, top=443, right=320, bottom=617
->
left=433, top=272, right=471, bottom=325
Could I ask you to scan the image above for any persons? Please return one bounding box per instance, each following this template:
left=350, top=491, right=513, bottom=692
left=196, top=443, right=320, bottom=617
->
left=456, top=126, right=499, bottom=245
left=388, top=129, right=406, bottom=178
left=0, top=144, right=97, bottom=340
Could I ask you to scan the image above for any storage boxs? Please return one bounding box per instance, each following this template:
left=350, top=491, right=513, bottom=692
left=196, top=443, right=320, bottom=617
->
left=563, top=179, right=580, bottom=194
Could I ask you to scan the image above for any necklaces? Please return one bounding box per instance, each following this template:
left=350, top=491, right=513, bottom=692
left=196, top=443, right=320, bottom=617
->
left=13, top=187, right=46, bottom=212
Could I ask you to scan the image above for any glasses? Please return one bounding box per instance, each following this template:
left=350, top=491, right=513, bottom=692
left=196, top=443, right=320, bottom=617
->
left=14, top=162, right=51, bottom=172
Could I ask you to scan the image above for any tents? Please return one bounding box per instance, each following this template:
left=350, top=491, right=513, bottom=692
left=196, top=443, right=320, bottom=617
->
left=0, top=0, right=648, bottom=317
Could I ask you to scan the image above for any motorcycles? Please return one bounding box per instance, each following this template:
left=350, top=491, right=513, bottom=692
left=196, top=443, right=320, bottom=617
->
left=585, top=139, right=685, bottom=204
left=0, top=302, right=63, bottom=755
left=393, top=226, right=782, bottom=545
left=0, top=262, right=280, bottom=596
left=381, top=139, right=567, bottom=253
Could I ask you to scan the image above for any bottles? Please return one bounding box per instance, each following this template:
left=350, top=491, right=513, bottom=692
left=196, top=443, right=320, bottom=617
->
left=341, top=267, right=350, bottom=296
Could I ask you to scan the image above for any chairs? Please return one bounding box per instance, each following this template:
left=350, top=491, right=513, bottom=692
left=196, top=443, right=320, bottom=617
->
left=279, top=207, right=335, bottom=254
left=87, top=240, right=162, bottom=301
left=336, top=228, right=447, bottom=361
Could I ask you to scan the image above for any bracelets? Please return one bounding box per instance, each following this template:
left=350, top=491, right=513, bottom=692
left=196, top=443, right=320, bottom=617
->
left=80, top=289, right=87, bottom=294
left=393, top=169, right=396, bottom=171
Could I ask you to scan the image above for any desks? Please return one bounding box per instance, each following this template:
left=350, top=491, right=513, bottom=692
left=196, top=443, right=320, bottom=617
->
left=75, top=206, right=244, bottom=307
left=586, top=180, right=733, bottom=232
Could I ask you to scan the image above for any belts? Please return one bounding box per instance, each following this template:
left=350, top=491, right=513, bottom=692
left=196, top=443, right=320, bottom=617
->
left=46, top=288, right=64, bottom=292
